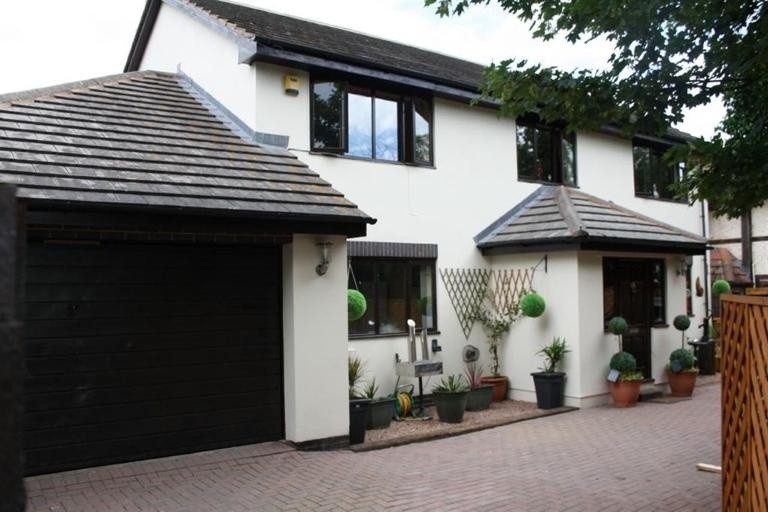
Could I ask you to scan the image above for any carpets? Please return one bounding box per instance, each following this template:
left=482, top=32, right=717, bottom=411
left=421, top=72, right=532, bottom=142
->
left=648, top=394, right=693, bottom=404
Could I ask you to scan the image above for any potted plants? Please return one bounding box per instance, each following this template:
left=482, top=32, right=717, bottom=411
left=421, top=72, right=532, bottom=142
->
left=667, top=315, right=699, bottom=397
left=530, top=336, right=572, bottom=410
left=431, top=332, right=508, bottom=423
left=347, top=354, right=396, bottom=445
left=608, top=317, right=644, bottom=407
